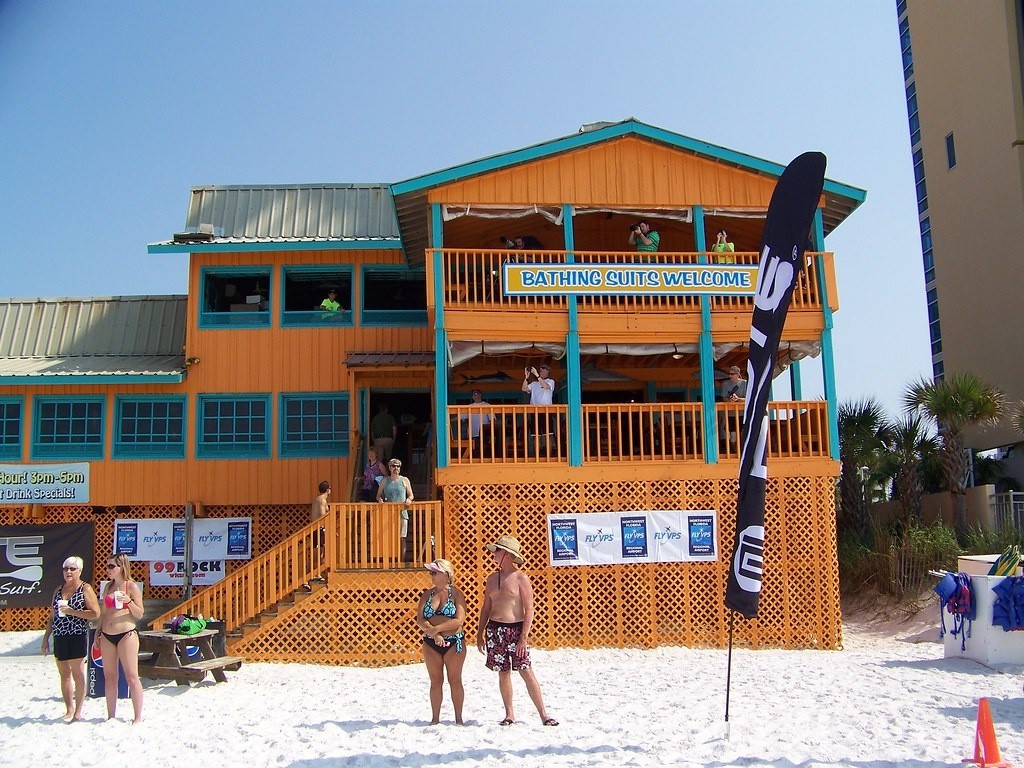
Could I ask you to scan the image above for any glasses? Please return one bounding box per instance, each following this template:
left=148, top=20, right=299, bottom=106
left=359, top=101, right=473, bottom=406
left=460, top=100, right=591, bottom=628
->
left=106, top=564, right=120, bottom=569
left=328, top=487, right=332, bottom=490
left=539, top=367, right=549, bottom=370
left=728, top=372, right=739, bottom=375
left=429, top=570, right=443, bottom=576
left=62, top=567, right=79, bottom=571
left=390, top=465, right=400, bottom=468
left=495, top=547, right=504, bottom=551
left=471, top=392, right=482, bottom=396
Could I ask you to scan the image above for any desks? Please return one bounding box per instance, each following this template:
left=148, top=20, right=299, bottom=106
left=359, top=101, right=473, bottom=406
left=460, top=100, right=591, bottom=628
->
left=671, top=419, right=700, bottom=453
left=770, top=419, right=786, bottom=452
left=584, top=423, right=607, bottom=456
left=482, top=423, right=518, bottom=458
left=139, top=627, right=229, bottom=686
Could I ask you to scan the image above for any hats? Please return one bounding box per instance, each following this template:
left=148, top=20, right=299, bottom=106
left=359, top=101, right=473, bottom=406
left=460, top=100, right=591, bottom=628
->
left=486, top=535, right=524, bottom=565
left=424, top=559, right=454, bottom=577
left=329, top=288, right=336, bottom=294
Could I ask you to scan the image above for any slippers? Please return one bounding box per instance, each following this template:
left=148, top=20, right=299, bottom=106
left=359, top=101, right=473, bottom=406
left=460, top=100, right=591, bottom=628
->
left=543, top=718, right=558, bottom=726
left=499, top=718, right=515, bottom=727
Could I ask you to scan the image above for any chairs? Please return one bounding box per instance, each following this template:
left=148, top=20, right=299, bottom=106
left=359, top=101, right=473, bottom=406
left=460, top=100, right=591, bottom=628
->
left=449, top=413, right=824, bottom=458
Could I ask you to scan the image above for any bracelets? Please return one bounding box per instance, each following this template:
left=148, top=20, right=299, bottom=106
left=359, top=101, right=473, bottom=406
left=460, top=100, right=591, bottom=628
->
left=639, top=232, right=643, bottom=235
left=408, top=497, right=412, bottom=501
left=127, top=601, right=132, bottom=604
left=537, top=376, right=540, bottom=379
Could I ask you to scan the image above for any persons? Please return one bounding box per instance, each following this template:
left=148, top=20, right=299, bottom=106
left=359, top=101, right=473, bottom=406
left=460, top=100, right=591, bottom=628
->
left=721, top=366, right=747, bottom=453
left=711, top=229, right=734, bottom=263
left=371, top=402, right=397, bottom=467
left=41, top=556, right=101, bottom=725
left=450, top=390, right=497, bottom=457
left=422, top=412, right=433, bottom=490
left=522, top=365, right=555, bottom=457
left=477, top=535, right=559, bottom=726
left=301, top=480, right=339, bottom=590
left=628, top=220, right=659, bottom=262
left=94, top=553, right=144, bottom=725
left=417, top=559, right=467, bottom=726
left=376, top=458, right=414, bottom=562
left=320, top=289, right=345, bottom=322
left=506, top=236, right=533, bottom=263
left=353, top=446, right=389, bottom=526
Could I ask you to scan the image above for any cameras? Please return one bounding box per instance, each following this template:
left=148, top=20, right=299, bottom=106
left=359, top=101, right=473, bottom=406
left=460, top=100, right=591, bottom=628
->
left=728, top=385, right=739, bottom=396
left=527, top=367, right=532, bottom=371
left=630, top=224, right=637, bottom=231
left=501, top=237, right=516, bottom=248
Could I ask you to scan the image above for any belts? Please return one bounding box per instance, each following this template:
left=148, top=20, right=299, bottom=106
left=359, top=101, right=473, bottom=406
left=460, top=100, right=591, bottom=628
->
left=375, top=436, right=391, bottom=438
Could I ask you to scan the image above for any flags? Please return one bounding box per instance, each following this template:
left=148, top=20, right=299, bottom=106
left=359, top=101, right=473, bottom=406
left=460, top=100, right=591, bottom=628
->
left=723, top=151, right=827, bottom=619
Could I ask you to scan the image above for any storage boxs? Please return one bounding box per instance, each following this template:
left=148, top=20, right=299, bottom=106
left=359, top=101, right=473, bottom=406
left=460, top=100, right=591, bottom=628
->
left=230, top=294, right=269, bottom=311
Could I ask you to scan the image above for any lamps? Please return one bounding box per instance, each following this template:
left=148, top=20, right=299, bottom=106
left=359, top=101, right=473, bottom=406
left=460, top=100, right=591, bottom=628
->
left=186, top=356, right=201, bottom=366
left=673, top=343, right=684, bottom=360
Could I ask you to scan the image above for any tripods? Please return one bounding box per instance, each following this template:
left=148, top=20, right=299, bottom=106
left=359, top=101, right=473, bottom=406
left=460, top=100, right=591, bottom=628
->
left=487, top=258, right=515, bottom=301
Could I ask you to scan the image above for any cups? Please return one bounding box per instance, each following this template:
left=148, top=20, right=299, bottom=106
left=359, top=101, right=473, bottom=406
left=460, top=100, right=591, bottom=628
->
left=114, top=590, right=125, bottom=609
left=57, top=599, right=69, bottom=617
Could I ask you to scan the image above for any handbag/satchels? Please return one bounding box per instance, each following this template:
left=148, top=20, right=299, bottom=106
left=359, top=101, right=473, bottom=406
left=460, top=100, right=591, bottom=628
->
left=170, top=613, right=206, bottom=635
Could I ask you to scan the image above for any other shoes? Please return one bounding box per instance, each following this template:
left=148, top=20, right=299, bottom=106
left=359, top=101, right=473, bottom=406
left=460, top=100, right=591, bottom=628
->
left=303, top=584, right=311, bottom=589
left=312, top=576, right=325, bottom=581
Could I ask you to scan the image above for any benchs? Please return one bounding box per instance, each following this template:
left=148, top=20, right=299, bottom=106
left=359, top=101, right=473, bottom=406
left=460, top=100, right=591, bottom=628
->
left=178, top=656, right=245, bottom=672
left=138, top=652, right=154, bottom=660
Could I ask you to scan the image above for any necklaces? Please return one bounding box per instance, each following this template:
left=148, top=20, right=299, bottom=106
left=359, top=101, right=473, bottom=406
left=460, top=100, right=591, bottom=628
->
left=370, top=460, right=378, bottom=467
